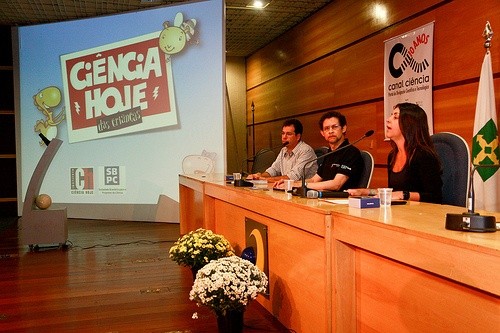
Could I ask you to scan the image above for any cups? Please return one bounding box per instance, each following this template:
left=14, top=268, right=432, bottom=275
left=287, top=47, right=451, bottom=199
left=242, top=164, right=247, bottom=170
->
left=283, top=180, right=295, bottom=194
left=233, top=173, right=241, bottom=180
left=378, top=188, right=393, bottom=207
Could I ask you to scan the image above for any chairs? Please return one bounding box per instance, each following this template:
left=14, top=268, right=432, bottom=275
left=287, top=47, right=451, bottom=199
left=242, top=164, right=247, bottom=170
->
left=431, top=132, right=471, bottom=208
left=252, top=148, right=278, bottom=174
left=316, top=146, right=329, bottom=165
left=359, top=151, right=374, bottom=188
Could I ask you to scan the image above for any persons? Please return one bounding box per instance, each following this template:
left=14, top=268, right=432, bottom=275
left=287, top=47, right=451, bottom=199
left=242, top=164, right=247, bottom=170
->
left=272, top=111, right=365, bottom=192
left=245, top=118, right=318, bottom=183
left=343, top=101, right=444, bottom=204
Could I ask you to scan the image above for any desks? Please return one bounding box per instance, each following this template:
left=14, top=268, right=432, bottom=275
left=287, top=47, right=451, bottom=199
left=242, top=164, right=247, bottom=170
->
left=178, top=174, right=500, bottom=333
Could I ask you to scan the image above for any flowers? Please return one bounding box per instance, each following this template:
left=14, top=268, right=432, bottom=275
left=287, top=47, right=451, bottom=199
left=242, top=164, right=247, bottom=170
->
left=169, top=227, right=236, bottom=269
left=189, top=256, right=270, bottom=320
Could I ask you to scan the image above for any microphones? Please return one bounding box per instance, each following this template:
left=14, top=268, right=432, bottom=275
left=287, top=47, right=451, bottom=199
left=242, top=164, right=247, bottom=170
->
left=234, top=141, right=290, bottom=187
left=291, top=130, right=375, bottom=199
left=306, top=190, right=350, bottom=199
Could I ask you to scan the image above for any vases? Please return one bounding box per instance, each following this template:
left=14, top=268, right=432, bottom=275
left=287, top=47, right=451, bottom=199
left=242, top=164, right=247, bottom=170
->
left=192, top=265, right=203, bottom=281
left=217, top=310, right=243, bottom=333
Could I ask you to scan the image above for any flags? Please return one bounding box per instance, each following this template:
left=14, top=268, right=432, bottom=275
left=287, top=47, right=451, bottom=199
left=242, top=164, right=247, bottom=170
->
left=466, top=50, right=500, bottom=213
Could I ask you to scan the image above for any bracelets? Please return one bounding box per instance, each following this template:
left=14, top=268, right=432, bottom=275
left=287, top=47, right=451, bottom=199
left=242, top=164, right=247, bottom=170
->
left=368, top=189, right=377, bottom=196
left=304, top=181, right=307, bottom=186
left=403, top=190, right=410, bottom=200
